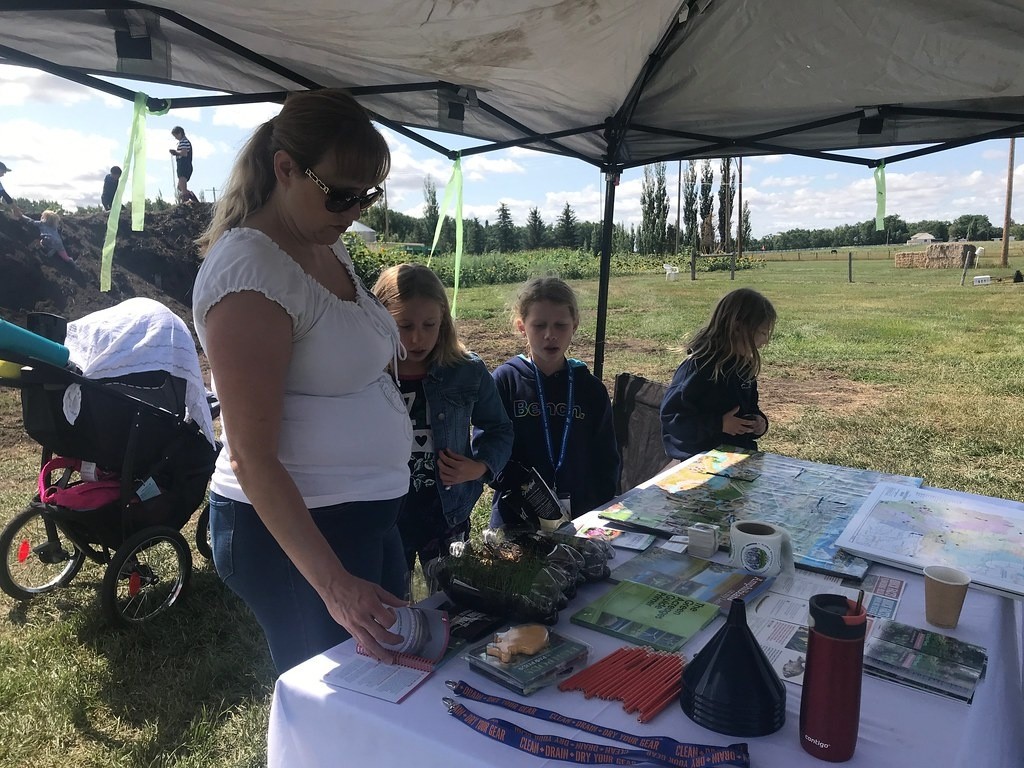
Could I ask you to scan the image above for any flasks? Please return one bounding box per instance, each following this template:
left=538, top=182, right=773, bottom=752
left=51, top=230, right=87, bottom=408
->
left=799, top=593, right=867, bottom=763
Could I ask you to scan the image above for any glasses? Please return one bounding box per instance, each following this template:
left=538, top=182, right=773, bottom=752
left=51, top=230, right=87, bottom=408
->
left=286, top=148, right=384, bottom=213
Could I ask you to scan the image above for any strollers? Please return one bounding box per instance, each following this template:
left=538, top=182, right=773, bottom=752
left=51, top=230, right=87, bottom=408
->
left=1, top=306, right=218, bottom=630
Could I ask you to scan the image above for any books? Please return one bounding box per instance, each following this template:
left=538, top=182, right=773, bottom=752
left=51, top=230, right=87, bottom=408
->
left=459, top=627, right=590, bottom=697
left=569, top=578, right=721, bottom=652
left=499, top=464, right=575, bottom=542
left=320, top=601, right=469, bottom=704
left=604, top=545, right=774, bottom=615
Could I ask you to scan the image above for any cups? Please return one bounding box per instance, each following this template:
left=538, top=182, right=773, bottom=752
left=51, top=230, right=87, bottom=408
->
left=923, top=565, right=971, bottom=629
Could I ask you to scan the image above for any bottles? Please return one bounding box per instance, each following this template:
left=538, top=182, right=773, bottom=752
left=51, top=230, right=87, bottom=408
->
left=478, top=522, right=616, bottom=584
left=423, top=556, right=573, bottom=622
left=448, top=537, right=585, bottom=599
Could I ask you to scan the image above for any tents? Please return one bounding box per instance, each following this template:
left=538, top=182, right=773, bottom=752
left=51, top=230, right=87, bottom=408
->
left=0, top=0, right=1023, bottom=382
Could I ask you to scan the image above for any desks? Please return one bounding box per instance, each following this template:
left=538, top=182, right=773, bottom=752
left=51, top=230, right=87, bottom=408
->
left=267, top=444, right=1024, bottom=768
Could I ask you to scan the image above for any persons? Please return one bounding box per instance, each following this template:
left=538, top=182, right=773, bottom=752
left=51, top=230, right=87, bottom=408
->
left=470, top=276, right=620, bottom=554
left=192, top=86, right=417, bottom=673
left=166, top=126, right=195, bottom=202
left=101, top=166, right=128, bottom=212
left=369, top=263, right=515, bottom=606
left=19, top=210, right=76, bottom=268
left=0, top=162, right=24, bottom=217
left=661, top=289, right=778, bottom=464
